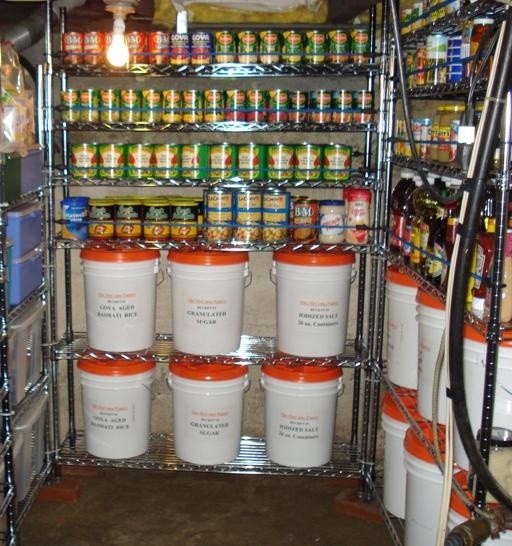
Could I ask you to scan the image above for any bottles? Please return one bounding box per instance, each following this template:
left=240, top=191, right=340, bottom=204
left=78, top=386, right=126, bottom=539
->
left=141, top=87, right=162, bottom=122
left=162, top=88, right=182, bottom=122
left=79, top=87, right=99, bottom=122
left=401, top=0, right=508, bottom=90
left=343, top=187, right=351, bottom=233
left=166, top=195, right=182, bottom=199
left=259, top=30, right=282, bottom=64
left=214, top=30, right=237, bottom=62
left=203, top=188, right=233, bottom=242
left=104, top=31, right=125, bottom=64
left=233, top=187, right=262, bottom=241
left=281, top=30, right=306, bottom=64
left=169, top=199, right=198, bottom=240
left=203, top=88, right=224, bottom=121
left=98, top=142, right=125, bottom=179
left=293, top=199, right=319, bottom=243
left=143, top=199, right=170, bottom=239
left=59, top=88, right=79, bottom=122
left=290, top=194, right=309, bottom=240
left=306, top=30, right=327, bottom=64
left=347, top=189, right=371, bottom=244
left=267, top=142, right=293, bottom=180
left=89, top=198, right=115, bottom=239
left=289, top=88, right=309, bottom=123
left=323, top=142, right=352, bottom=181
left=309, top=88, right=332, bottom=124
left=328, top=29, right=350, bottom=64
left=124, top=142, right=153, bottom=179
left=143, top=195, right=167, bottom=199
left=148, top=31, right=170, bottom=65
left=120, top=88, right=140, bottom=122
left=209, top=142, right=237, bottom=179
left=104, top=195, right=143, bottom=198
left=353, top=89, right=374, bottom=124
left=115, top=198, right=143, bottom=239
left=393, top=106, right=501, bottom=169
left=125, top=31, right=148, bottom=63
left=350, top=29, right=371, bottom=64
left=262, top=186, right=290, bottom=242
left=182, top=196, right=204, bottom=236
left=245, top=88, right=266, bottom=122
left=319, top=199, right=345, bottom=243
left=84, top=31, right=104, bottom=64
left=62, top=31, right=84, bottom=64
left=266, top=88, right=289, bottom=122
left=153, top=142, right=181, bottom=178
left=237, top=142, right=266, bottom=181
left=332, top=89, right=353, bottom=124
left=389, top=173, right=512, bottom=324
left=100, top=88, right=120, bottom=121
left=181, top=142, right=209, bottom=180
left=60, top=196, right=89, bottom=241
left=237, top=30, right=259, bottom=63
left=191, top=31, right=214, bottom=64
left=292, top=142, right=323, bottom=181
left=224, top=87, right=245, bottom=121
left=71, top=142, right=98, bottom=178
left=170, top=32, right=191, bottom=64
left=181, top=88, right=203, bottom=122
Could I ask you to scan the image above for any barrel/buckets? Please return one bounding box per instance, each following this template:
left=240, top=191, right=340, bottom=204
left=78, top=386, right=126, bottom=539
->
left=385, top=263, right=420, bottom=391
left=448, top=470, right=511, bottom=546
left=166, top=353, right=252, bottom=468
left=167, top=248, right=252, bottom=357
left=259, top=353, right=345, bottom=470
left=452, top=464, right=465, bottom=476
left=76, top=353, right=157, bottom=460
left=402, top=422, right=448, bottom=545
left=453, top=318, right=512, bottom=505
left=80, top=247, right=164, bottom=354
left=270, top=247, right=357, bottom=359
left=415, top=286, right=451, bottom=427
left=381, top=388, right=429, bottom=522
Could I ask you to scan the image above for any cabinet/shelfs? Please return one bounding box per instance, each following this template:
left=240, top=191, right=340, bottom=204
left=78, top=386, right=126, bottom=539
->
left=362, top=1, right=512, bottom=546
left=44, top=0, right=387, bottom=498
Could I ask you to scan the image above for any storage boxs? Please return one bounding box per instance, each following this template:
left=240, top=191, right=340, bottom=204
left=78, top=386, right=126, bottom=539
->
left=0, top=205, right=44, bottom=261
left=0, top=383, right=54, bottom=505
left=3, top=244, right=46, bottom=306
left=6, top=299, right=46, bottom=409
left=0, top=148, right=45, bottom=209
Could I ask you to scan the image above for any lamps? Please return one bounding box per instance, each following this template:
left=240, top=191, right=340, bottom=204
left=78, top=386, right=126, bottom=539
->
left=100, top=0, right=135, bottom=70
